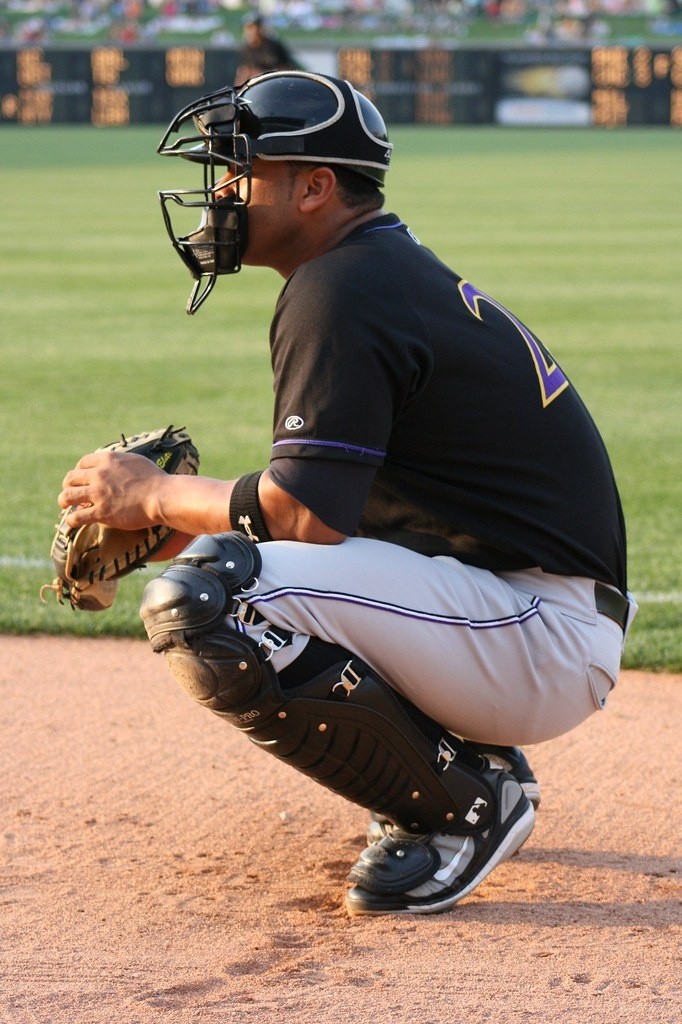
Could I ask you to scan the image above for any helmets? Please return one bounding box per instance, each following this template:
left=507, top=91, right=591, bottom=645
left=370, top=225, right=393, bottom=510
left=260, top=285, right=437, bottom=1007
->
left=177, top=69, right=390, bottom=187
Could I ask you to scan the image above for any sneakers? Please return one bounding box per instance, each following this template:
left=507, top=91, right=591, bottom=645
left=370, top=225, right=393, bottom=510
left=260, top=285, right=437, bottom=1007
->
left=345, top=740, right=540, bottom=916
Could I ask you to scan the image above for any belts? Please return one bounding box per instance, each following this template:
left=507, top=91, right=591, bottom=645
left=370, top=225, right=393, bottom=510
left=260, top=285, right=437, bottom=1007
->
left=594, top=583, right=627, bottom=631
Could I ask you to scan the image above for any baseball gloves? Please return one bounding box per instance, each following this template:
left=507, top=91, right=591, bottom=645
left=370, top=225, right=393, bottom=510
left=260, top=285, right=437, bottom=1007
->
left=50, top=427, right=200, bottom=612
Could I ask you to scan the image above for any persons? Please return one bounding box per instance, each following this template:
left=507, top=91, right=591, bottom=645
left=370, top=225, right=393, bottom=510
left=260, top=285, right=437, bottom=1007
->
left=37, top=69, right=638, bottom=918
left=234, top=12, right=303, bottom=94
